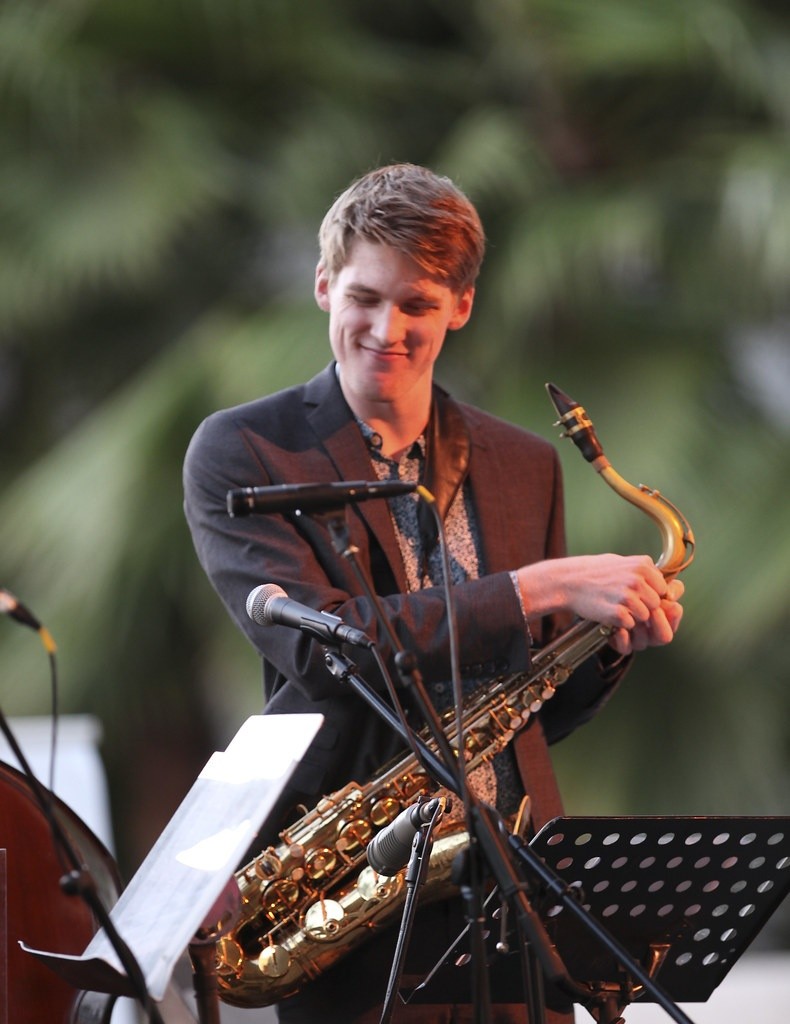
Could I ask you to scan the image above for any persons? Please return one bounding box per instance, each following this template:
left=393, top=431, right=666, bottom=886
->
left=183, top=162, right=686, bottom=1024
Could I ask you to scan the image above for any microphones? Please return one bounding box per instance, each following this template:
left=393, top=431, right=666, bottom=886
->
left=245, top=583, right=375, bottom=649
left=227, top=483, right=417, bottom=518
left=366, top=797, right=445, bottom=877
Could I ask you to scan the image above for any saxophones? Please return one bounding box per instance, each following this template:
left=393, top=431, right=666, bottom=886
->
left=204, top=378, right=695, bottom=1007
